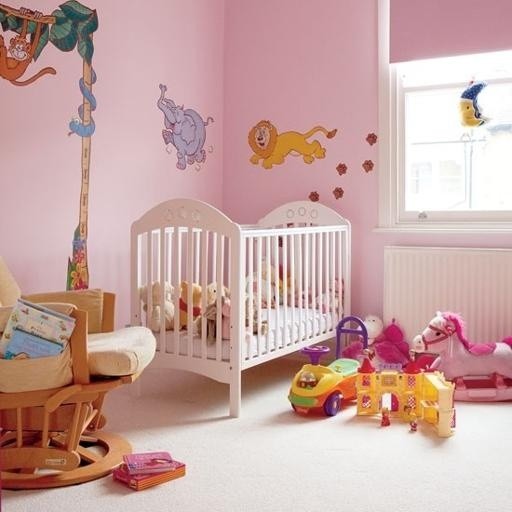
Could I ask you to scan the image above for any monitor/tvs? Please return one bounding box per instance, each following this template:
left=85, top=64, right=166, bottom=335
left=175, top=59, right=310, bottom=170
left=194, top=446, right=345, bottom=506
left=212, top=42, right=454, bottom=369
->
left=112, top=462, right=187, bottom=492
left=123, top=451, right=175, bottom=475
left=0, top=299, right=75, bottom=356
left=4, top=328, right=63, bottom=360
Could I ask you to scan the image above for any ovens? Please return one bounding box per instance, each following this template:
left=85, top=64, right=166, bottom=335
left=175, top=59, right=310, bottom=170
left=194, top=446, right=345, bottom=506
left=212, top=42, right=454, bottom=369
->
left=130, top=199, right=351, bottom=418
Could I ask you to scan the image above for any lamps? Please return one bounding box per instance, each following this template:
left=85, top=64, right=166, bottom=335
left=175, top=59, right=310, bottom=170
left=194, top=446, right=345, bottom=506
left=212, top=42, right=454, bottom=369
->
left=0, top=256, right=157, bottom=488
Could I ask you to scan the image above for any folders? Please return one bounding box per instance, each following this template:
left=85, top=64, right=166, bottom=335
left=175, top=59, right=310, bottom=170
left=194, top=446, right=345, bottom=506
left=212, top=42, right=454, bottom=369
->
left=383, top=245, right=511, bottom=354
